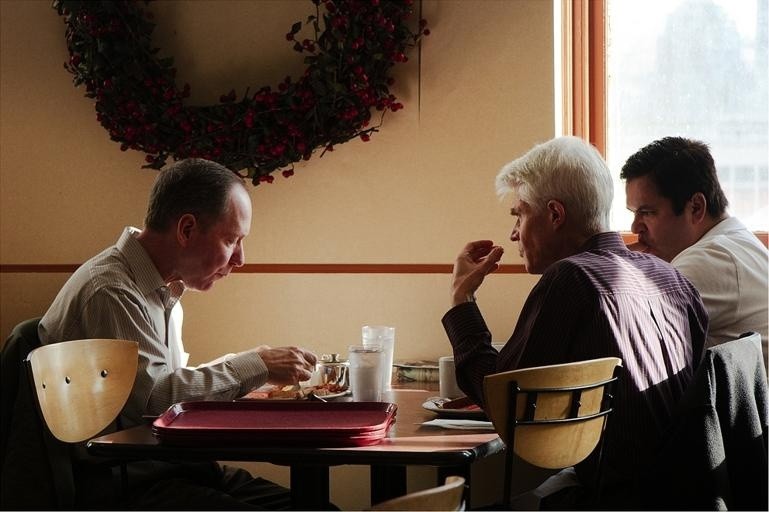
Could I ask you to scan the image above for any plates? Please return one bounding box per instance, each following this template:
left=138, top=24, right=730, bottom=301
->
left=239, top=390, right=347, bottom=401
left=422, top=398, right=484, bottom=413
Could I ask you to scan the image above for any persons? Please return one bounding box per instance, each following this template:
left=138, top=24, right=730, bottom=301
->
left=441, top=133, right=708, bottom=510
left=620, top=136, right=768, bottom=378
left=37, top=157, right=341, bottom=511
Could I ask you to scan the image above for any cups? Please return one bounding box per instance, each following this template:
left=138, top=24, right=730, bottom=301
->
left=439, top=357, right=467, bottom=398
left=348, top=325, right=394, bottom=402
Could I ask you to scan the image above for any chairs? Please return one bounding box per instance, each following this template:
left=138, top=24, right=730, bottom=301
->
left=677, top=329, right=765, bottom=512
left=364, top=475, right=469, bottom=511
left=10, top=314, right=139, bottom=512
left=480, top=354, right=624, bottom=510
